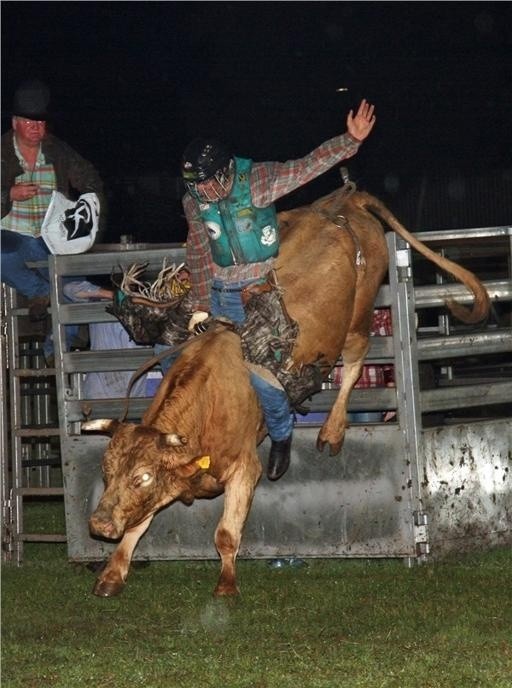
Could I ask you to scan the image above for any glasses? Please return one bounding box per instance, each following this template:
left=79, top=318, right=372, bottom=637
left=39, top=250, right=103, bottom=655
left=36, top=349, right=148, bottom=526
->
left=21, top=120, right=47, bottom=128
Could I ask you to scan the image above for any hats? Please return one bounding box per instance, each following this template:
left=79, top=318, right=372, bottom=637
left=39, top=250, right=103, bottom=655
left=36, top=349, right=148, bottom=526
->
left=11, top=100, right=51, bottom=122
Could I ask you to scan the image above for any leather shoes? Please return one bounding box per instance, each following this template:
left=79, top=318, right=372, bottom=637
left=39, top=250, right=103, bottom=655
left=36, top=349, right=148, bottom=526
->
left=266, top=429, right=293, bottom=482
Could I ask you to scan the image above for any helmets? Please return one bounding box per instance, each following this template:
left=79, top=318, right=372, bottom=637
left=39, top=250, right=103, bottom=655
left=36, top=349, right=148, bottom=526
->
left=179, top=133, right=237, bottom=206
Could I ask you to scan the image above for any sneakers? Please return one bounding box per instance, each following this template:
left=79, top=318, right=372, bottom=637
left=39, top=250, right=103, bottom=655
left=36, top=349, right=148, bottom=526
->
left=26, top=293, right=51, bottom=323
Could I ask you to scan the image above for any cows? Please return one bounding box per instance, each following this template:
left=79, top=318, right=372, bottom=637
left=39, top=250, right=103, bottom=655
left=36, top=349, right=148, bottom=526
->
left=79, top=178, right=492, bottom=601
